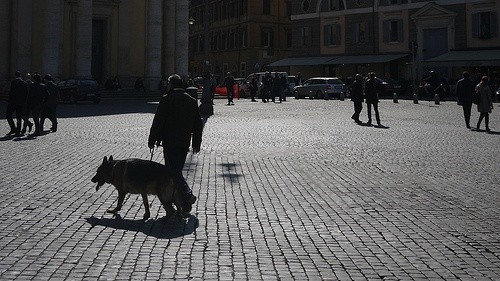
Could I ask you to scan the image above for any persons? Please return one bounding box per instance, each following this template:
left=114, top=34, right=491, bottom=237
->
left=210, top=74, right=217, bottom=105
left=350, top=73, right=365, bottom=125
left=5, top=72, right=59, bottom=137
left=364, top=72, right=382, bottom=127
left=423, top=70, right=437, bottom=98
left=475, top=76, right=494, bottom=132
left=249, top=71, right=303, bottom=104
left=147, top=72, right=203, bottom=218
left=224, top=71, right=234, bottom=105
left=454, top=71, right=475, bottom=129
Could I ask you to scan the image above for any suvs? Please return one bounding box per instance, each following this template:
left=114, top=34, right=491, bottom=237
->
left=293, top=76, right=346, bottom=99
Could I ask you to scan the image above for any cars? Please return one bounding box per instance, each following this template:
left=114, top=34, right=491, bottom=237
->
left=216, top=77, right=246, bottom=97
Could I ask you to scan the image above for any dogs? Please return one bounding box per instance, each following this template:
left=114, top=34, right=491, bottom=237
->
left=91, top=155, right=183, bottom=224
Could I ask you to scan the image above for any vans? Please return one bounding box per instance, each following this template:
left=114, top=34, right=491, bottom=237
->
left=241, top=72, right=289, bottom=98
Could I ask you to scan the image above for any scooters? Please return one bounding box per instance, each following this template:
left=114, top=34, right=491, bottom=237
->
left=416, top=80, right=447, bottom=101
